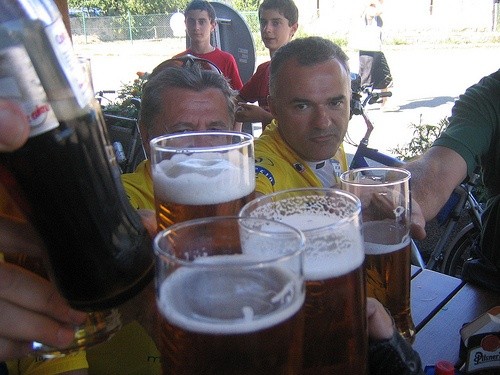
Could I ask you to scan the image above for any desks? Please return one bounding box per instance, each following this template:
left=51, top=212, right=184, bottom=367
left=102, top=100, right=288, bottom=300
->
left=410, top=263, right=500, bottom=375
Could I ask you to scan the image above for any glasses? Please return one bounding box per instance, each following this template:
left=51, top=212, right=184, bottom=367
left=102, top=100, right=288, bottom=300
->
left=148, top=56, right=224, bottom=82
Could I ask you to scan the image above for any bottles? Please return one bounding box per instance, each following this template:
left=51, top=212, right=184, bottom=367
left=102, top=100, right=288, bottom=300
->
left=0, top=0, right=159, bottom=312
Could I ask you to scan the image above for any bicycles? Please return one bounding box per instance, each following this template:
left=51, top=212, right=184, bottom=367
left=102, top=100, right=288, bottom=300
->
left=349, top=88, right=483, bottom=277
left=94, top=90, right=143, bottom=175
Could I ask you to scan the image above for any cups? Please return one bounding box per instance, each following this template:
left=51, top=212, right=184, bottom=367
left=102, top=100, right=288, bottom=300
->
left=32, top=307, right=124, bottom=355
left=338, top=166, right=413, bottom=321
left=152, top=216, right=304, bottom=375
left=149, top=131, right=256, bottom=303
left=237, top=187, right=368, bottom=375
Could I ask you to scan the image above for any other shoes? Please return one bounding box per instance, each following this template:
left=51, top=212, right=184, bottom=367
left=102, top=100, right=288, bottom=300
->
left=379, top=104, right=400, bottom=112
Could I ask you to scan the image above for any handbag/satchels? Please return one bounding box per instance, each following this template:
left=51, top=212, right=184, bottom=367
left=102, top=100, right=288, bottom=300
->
left=352, top=8, right=381, bottom=51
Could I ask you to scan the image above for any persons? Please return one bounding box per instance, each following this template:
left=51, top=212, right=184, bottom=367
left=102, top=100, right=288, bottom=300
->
left=244, top=35, right=394, bottom=340
left=322, top=1, right=500, bottom=291
left=171, top=0, right=243, bottom=91
left=235, top=0, right=298, bottom=131
left=0, top=55, right=239, bottom=375
